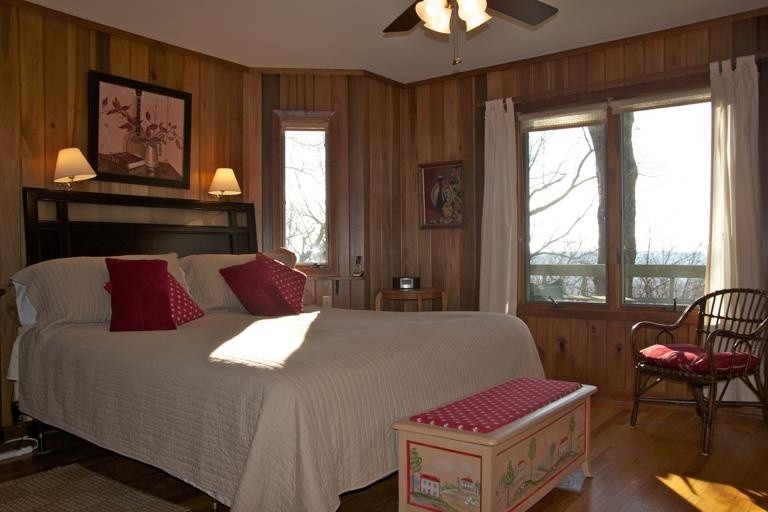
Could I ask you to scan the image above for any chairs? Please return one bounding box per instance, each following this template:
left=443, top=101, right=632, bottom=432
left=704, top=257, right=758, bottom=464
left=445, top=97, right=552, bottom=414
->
left=626, top=283, right=768, bottom=459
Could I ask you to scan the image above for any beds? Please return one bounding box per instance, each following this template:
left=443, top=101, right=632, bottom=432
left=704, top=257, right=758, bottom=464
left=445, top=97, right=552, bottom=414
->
left=5, top=184, right=549, bottom=512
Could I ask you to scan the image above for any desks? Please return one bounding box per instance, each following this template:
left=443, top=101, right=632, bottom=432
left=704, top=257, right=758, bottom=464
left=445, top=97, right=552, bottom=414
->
left=367, top=287, right=451, bottom=314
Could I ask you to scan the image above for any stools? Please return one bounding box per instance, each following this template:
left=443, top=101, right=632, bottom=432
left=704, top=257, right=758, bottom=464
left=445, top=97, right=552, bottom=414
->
left=387, top=371, right=603, bottom=510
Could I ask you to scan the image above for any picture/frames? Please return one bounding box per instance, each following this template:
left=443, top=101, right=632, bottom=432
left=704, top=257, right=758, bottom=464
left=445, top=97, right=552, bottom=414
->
left=417, top=159, right=467, bottom=231
left=87, top=72, right=194, bottom=190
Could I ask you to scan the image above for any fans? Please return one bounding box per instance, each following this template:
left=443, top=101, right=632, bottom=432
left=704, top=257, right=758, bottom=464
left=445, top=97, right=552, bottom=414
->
left=378, top=1, right=561, bottom=37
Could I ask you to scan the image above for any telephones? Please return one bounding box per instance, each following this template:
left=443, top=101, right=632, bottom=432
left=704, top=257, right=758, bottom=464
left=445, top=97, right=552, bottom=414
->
left=353, top=256, right=362, bottom=276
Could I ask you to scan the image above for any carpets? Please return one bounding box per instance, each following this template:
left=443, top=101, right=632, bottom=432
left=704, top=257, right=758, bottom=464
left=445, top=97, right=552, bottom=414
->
left=0, top=457, right=193, bottom=510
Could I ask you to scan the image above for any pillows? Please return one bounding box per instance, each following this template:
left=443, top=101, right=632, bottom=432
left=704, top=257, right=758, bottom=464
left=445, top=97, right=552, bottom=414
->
left=216, top=257, right=300, bottom=320
left=252, top=248, right=308, bottom=315
left=179, top=247, right=256, bottom=315
left=100, top=270, right=207, bottom=325
left=103, top=252, right=179, bottom=336
left=3, top=245, right=192, bottom=334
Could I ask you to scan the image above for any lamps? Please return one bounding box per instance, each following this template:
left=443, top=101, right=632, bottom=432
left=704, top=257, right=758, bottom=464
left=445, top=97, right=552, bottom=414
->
left=414, top=1, right=496, bottom=67
left=206, top=167, right=240, bottom=201
left=47, top=146, right=97, bottom=190
